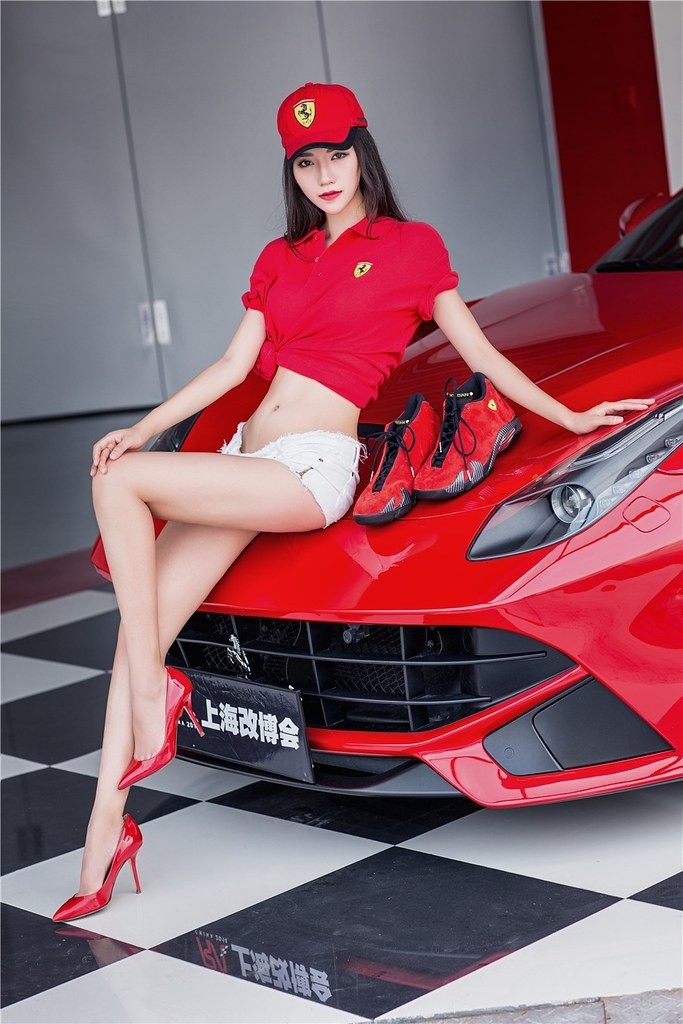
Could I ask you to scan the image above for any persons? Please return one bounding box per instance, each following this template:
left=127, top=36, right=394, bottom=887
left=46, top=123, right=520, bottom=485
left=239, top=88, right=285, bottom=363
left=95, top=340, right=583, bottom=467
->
left=50, top=80, right=657, bottom=924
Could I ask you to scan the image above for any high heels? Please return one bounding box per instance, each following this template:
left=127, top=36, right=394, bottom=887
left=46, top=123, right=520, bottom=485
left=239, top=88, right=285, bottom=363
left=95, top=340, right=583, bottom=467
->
left=53, top=813, right=143, bottom=923
left=118, top=665, right=205, bottom=789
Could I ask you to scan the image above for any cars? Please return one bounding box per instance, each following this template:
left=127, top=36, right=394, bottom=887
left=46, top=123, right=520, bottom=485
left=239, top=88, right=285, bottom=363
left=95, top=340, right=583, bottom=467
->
left=81, top=186, right=683, bottom=809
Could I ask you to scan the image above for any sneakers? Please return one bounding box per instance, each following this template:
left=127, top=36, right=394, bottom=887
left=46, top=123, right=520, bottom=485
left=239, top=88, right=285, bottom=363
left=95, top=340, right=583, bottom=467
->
left=413, top=372, right=520, bottom=500
left=353, top=393, right=440, bottom=526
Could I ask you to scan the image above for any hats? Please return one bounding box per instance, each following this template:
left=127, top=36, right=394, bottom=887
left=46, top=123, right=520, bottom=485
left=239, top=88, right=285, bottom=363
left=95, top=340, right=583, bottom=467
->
left=277, top=82, right=368, bottom=160
